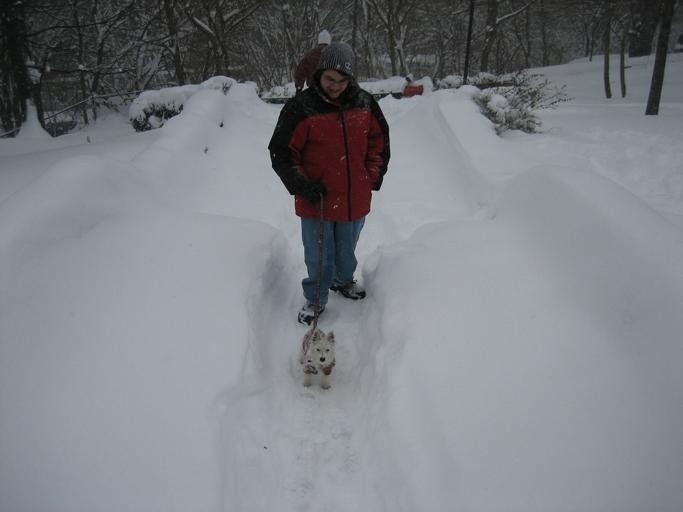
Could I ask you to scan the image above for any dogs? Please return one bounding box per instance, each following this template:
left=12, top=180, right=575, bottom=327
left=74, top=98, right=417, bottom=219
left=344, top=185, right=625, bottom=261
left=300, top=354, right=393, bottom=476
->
left=299, top=326, right=335, bottom=389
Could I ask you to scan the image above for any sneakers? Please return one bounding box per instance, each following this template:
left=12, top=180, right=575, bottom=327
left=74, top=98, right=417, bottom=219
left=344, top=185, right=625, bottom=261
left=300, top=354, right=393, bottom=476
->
left=298, top=302, right=325, bottom=324
left=330, top=281, right=366, bottom=299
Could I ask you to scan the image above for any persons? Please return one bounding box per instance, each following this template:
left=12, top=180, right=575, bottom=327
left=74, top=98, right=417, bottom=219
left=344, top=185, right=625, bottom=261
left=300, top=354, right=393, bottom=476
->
left=268, top=41, right=390, bottom=325
left=294, top=29, right=332, bottom=96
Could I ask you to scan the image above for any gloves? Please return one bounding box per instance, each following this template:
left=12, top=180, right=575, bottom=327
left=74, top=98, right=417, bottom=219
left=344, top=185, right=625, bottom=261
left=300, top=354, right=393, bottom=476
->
left=302, top=182, right=327, bottom=202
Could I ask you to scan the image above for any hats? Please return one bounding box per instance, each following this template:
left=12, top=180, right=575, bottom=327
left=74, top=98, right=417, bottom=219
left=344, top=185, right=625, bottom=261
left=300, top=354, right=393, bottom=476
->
left=318, top=30, right=331, bottom=45
left=318, top=42, right=355, bottom=76
